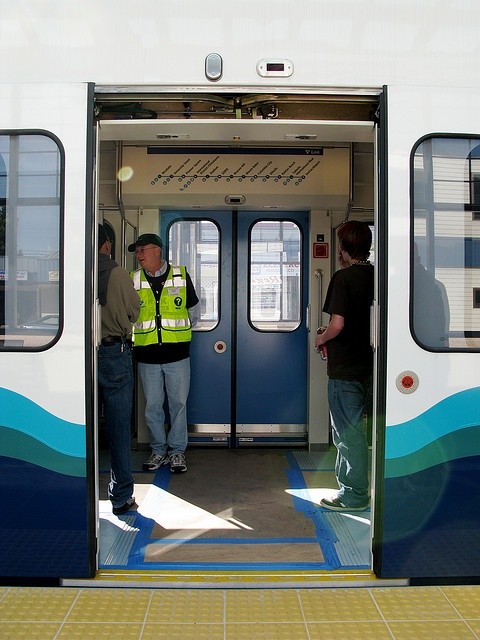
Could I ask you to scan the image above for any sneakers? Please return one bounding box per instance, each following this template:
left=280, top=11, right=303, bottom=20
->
left=113, top=496, right=135, bottom=515
left=320, top=493, right=369, bottom=512
left=168, top=453, right=188, bottom=474
left=142, top=453, right=170, bottom=473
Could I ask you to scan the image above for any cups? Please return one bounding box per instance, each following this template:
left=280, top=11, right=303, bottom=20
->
left=317, top=325, right=328, bottom=360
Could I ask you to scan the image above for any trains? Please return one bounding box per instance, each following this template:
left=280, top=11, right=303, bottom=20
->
left=0, top=2, right=479, bottom=587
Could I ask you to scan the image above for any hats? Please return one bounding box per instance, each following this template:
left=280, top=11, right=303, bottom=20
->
left=128, top=234, right=163, bottom=252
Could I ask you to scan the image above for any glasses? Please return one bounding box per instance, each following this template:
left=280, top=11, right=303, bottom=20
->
left=134, top=246, right=157, bottom=255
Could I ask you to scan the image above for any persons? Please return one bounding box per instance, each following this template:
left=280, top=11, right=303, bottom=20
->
left=415, top=244, right=450, bottom=349
left=314, top=221, right=371, bottom=511
left=128, top=233, right=199, bottom=472
left=97, top=221, right=141, bottom=516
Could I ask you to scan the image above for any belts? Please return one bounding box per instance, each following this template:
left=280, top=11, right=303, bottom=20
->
left=102, top=336, right=130, bottom=343
